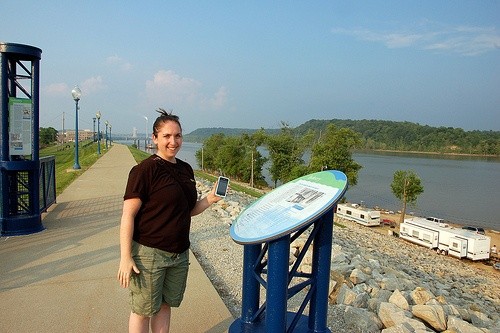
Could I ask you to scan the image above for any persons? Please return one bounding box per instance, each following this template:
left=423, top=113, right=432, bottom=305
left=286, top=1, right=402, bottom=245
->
left=491, top=245, right=497, bottom=254
left=118, top=108, right=230, bottom=333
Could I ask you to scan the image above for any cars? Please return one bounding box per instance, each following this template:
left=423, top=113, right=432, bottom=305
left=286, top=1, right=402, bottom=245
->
left=426, top=218, right=449, bottom=228
left=462, top=226, right=485, bottom=236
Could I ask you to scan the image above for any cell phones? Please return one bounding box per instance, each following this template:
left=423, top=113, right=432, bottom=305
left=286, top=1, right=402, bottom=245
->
left=215, top=176, right=230, bottom=197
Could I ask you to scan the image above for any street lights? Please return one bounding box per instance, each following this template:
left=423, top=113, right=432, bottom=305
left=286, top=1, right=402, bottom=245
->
left=96, top=112, right=101, bottom=154
left=93, top=118, right=96, bottom=142
left=71, top=84, right=82, bottom=169
left=105, top=120, right=109, bottom=149
left=109, top=125, right=112, bottom=146
